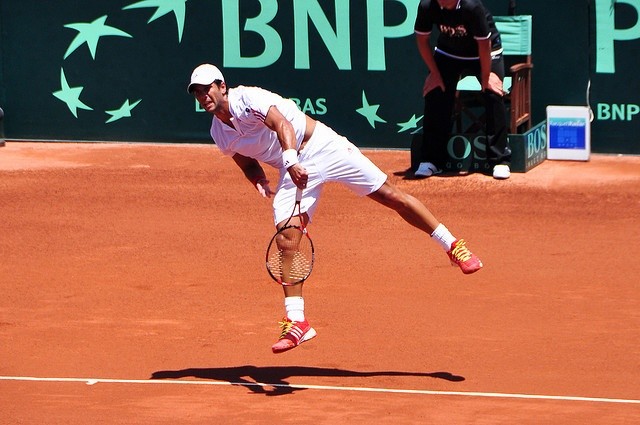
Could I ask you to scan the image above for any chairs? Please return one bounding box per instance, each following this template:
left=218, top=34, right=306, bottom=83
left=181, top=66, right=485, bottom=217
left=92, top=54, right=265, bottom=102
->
left=452, top=14, right=534, bottom=137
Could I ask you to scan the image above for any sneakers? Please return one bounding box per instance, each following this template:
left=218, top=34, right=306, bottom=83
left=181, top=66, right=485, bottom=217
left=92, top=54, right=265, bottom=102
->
left=415, top=161, right=442, bottom=178
left=492, top=164, right=511, bottom=179
left=271, top=315, right=317, bottom=354
left=446, top=238, right=483, bottom=274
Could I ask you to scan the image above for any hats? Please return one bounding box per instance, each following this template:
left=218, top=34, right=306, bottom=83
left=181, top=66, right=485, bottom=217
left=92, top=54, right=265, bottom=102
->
left=187, top=63, right=225, bottom=95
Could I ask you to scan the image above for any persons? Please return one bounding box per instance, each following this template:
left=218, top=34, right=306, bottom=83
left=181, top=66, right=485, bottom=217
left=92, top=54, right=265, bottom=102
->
left=187, top=64, right=482, bottom=353
left=415, top=0, right=511, bottom=179
